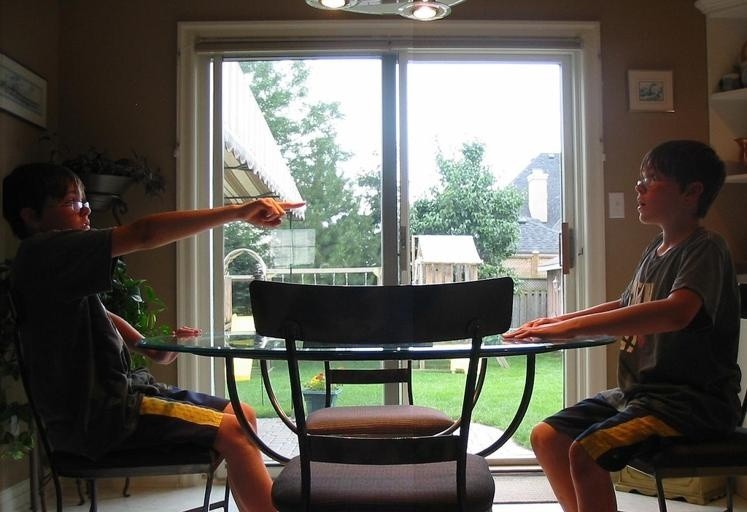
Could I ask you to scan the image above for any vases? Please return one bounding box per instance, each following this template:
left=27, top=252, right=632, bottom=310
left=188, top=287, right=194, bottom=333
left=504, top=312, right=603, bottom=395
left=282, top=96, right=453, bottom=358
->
left=301, top=391, right=338, bottom=416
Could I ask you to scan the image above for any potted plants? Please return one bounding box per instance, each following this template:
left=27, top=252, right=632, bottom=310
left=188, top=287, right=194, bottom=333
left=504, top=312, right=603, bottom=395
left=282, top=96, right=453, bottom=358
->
left=40, top=133, right=168, bottom=212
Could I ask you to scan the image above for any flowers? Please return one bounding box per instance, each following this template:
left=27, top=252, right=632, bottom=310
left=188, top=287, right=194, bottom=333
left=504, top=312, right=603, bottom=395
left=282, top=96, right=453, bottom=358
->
left=303, top=370, right=344, bottom=392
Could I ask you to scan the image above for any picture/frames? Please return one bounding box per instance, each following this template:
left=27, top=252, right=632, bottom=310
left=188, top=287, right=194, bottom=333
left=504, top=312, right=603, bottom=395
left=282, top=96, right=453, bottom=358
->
left=624, top=65, right=677, bottom=113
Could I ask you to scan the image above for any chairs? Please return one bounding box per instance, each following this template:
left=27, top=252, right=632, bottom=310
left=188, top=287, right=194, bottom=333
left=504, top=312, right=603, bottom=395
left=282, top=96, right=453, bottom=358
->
left=302, top=340, right=455, bottom=436
left=3, top=272, right=230, bottom=511
left=624, top=282, right=746, bottom=511
left=248, top=276, right=514, bottom=511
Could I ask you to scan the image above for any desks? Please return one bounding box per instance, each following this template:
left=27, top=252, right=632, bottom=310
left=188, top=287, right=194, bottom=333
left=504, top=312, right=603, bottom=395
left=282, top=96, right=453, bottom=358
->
left=134, top=329, right=619, bottom=512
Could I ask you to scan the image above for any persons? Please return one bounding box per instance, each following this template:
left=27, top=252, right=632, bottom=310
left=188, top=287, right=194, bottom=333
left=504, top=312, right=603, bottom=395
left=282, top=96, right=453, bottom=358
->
left=499, top=137, right=742, bottom=512
left=0, top=159, right=308, bottom=511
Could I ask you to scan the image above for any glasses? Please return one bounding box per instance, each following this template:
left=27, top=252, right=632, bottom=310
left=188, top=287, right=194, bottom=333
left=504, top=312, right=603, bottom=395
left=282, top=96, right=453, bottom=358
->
left=50, top=200, right=90, bottom=214
left=636, top=175, right=688, bottom=191
